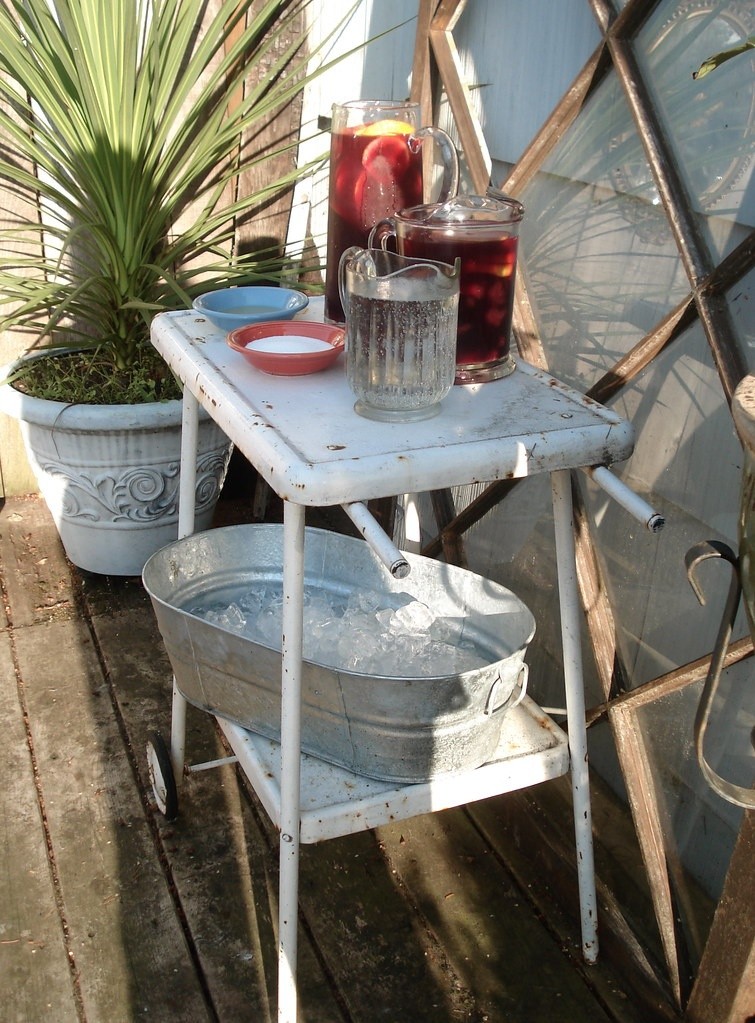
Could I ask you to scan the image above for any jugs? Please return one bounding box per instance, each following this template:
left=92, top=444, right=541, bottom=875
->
left=322, top=101, right=458, bottom=328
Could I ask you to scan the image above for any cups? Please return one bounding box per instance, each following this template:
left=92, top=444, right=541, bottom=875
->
left=338, top=245, right=461, bottom=423
left=368, top=195, right=517, bottom=384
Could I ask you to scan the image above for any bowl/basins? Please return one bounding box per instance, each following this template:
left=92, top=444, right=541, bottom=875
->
left=192, top=286, right=309, bottom=332
left=228, top=321, right=347, bottom=377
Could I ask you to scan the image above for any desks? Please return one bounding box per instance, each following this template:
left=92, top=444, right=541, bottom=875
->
left=146, top=280, right=636, bottom=1023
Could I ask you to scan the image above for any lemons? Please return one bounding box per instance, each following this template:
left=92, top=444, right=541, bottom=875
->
left=358, top=119, right=416, bottom=136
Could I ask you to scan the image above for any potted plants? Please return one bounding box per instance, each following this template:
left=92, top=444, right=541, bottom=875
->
left=1, top=0, right=501, bottom=576
